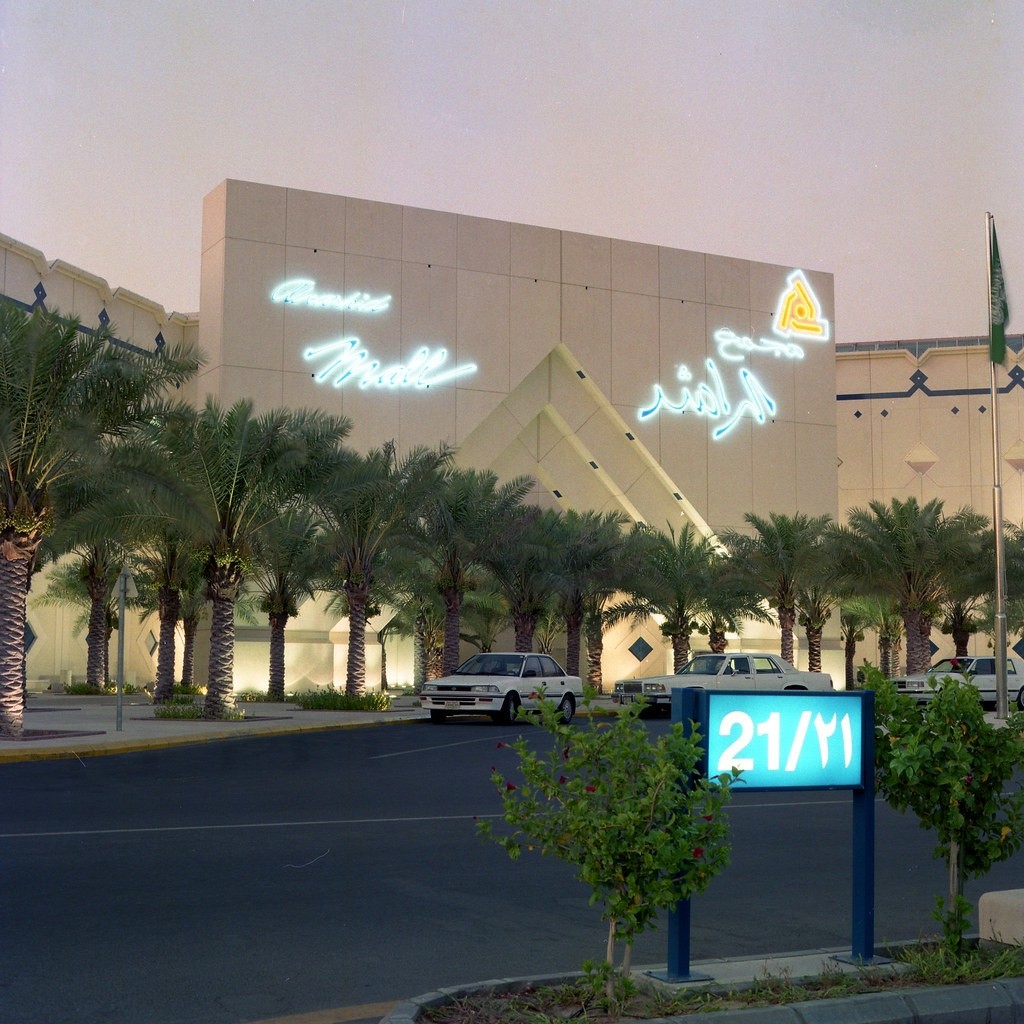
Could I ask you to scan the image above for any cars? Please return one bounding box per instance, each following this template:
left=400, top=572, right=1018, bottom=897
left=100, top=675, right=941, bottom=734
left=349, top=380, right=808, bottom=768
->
left=420, top=653, right=582, bottom=728
left=609, top=653, right=836, bottom=716
left=889, top=656, right=1024, bottom=711
